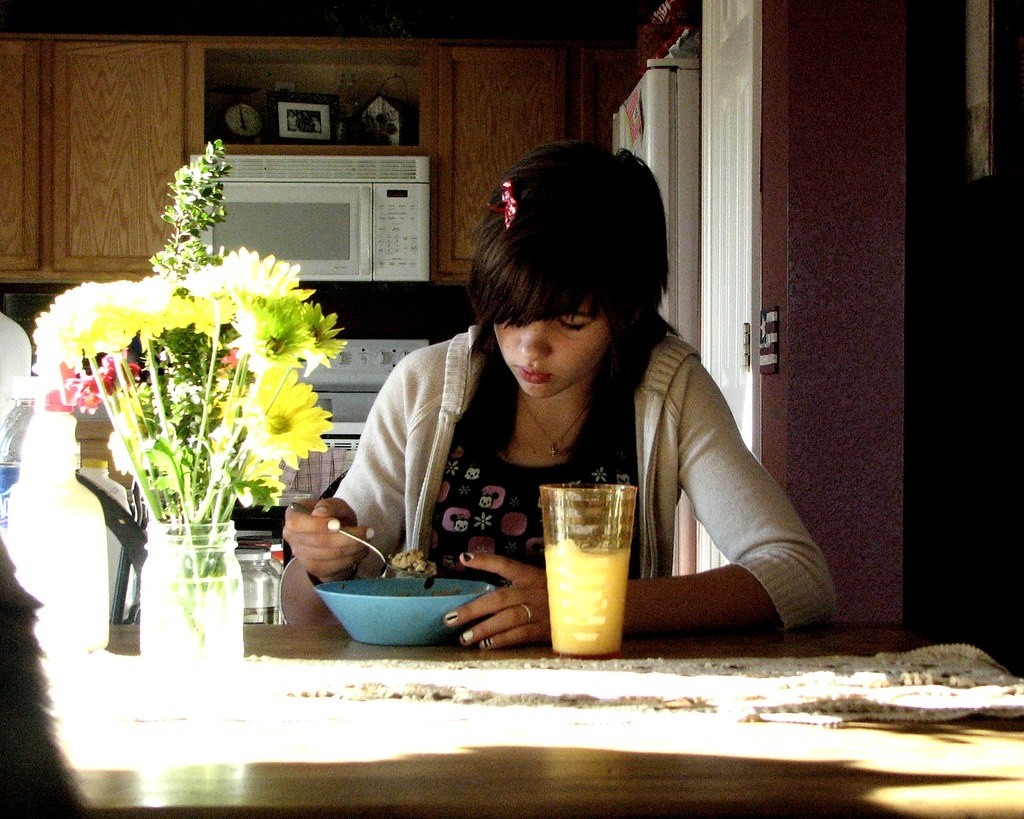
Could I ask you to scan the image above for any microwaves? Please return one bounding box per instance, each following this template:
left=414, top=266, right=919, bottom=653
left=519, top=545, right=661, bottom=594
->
left=189, top=154, right=431, bottom=281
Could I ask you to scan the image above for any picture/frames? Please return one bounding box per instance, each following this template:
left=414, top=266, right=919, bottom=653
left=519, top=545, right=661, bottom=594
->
left=273, top=93, right=338, bottom=144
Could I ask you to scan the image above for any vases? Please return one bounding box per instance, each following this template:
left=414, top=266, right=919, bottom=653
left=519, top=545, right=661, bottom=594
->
left=141, top=517, right=246, bottom=698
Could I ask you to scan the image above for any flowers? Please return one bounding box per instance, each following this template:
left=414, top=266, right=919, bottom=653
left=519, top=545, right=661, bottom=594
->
left=33, top=249, right=345, bottom=525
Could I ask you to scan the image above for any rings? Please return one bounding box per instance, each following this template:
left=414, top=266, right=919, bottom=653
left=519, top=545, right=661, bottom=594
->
left=522, top=604, right=533, bottom=623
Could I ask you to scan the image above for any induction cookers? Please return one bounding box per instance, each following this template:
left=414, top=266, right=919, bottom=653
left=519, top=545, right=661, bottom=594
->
left=295, top=338, right=430, bottom=448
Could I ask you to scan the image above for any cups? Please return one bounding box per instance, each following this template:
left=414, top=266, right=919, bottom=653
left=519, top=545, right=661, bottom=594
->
left=539, top=483, right=639, bottom=660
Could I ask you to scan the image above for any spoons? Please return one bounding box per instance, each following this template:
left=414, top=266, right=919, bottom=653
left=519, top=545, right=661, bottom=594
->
left=290, top=502, right=437, bottom=575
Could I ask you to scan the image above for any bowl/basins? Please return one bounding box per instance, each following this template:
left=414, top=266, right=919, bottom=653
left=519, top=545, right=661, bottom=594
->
left=312, top=577, right=496, bottom=646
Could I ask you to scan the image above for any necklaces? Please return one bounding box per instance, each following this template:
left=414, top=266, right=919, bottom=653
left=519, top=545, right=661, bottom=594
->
left=518, top=391, right=589, bottom=455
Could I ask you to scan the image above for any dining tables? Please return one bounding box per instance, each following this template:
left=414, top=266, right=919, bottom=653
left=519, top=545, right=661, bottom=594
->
left=0, top=626, right=1024, bottom=819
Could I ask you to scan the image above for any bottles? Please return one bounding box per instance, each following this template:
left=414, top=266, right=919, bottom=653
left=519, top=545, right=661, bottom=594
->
left=77, top=458, right=137, bottom=624
left=235, top=538, right=284, bottom=625
left=0, top=376, right=110, bottom=652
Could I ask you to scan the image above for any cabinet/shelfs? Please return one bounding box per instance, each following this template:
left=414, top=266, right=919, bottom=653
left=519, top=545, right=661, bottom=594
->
left=0, top=30, right=639, bottom=284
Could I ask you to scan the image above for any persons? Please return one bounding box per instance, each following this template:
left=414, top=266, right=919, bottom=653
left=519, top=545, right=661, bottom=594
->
left=0, top=314, right=33, bottom=420
left=277, top=137, right=834, bottom=650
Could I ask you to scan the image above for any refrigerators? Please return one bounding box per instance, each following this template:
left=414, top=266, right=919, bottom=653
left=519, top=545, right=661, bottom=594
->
left=611, top=56, right=704, bottom=576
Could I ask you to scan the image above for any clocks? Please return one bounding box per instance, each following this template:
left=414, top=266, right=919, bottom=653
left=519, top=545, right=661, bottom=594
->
left=224, top=103, right=264, bottom=140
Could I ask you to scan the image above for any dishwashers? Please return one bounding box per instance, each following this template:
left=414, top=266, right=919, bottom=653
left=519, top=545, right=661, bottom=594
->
left=264, top=451, right=377, bottom=505
